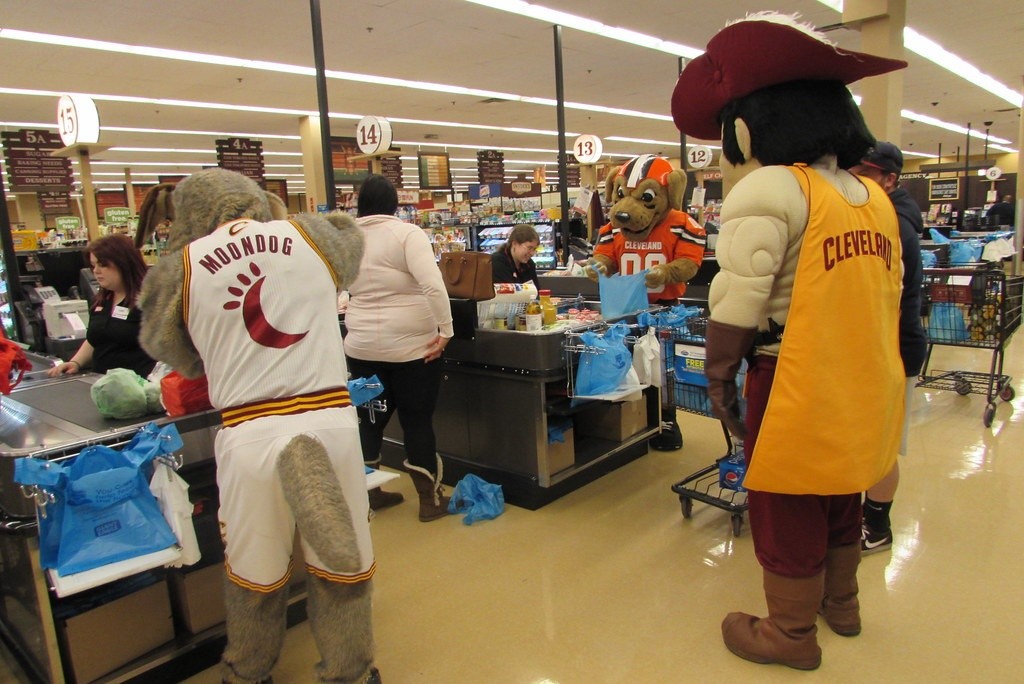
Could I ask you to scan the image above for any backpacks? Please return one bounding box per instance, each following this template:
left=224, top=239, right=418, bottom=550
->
left=591, top=264, right=648, bottom=318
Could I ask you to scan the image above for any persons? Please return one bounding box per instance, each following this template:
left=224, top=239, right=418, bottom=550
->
left=986, top=195, right=1014, bottom=226
left=336, top=173, right=469, bottom=520
left=47, top=234, right=157, bottom=379
left=491, top=225, right=540, bottom=291
left=849, top=140, right=926, bottom=554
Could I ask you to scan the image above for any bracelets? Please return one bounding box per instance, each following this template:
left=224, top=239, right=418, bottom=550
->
left=69, top=361, right=80, bottom=369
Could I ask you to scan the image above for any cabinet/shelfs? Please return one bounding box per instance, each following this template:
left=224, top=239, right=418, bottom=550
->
left=423, top=222, right=557, bottom=269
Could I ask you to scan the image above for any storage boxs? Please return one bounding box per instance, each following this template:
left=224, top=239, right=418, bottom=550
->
left=57, top=578, right=176, bottom=683
left=168, top=532, right=308, bottom=635
left=571, top=394, right=647, bottom=442
left=547, top=428, right=576, bottom=476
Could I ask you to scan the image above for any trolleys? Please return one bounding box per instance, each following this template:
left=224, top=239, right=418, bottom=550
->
left=658, top=315, right=749, bottom=537
left=914, top=261, right=1022, bottom=428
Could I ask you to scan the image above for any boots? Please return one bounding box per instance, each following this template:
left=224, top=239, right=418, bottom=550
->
left=365, top=453, right=404, bottom=509
left=403, top=452, right=464, bottom=521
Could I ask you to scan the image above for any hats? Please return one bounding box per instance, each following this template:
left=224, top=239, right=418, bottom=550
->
left=859, top=141, right=904, bottom=177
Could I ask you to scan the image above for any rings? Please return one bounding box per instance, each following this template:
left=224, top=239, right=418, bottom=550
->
left=442, top=348, right=445, bottom=352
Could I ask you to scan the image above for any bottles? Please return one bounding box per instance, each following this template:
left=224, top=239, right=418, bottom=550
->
left=525, top=296, right=542, bottom=332
left=540, top=291, right=556, bottom=325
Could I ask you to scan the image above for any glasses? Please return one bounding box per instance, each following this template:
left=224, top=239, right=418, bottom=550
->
left=520, top=243, right=537, bottom=254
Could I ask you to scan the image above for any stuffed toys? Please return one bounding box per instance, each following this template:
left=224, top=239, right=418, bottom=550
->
left=586, top=153, right=706, bottom=449
left=133, top=168, right=383, bottom=684
left=671, top=8, right=906, bottom=669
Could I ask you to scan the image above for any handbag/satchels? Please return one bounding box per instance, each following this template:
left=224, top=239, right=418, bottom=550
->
left=440, top=251, right=497, bottom=302
left=574, top=321, right=632, bottom=396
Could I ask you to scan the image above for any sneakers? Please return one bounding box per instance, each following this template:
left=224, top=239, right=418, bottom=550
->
left=858, top=522, right=893, bottom=556
